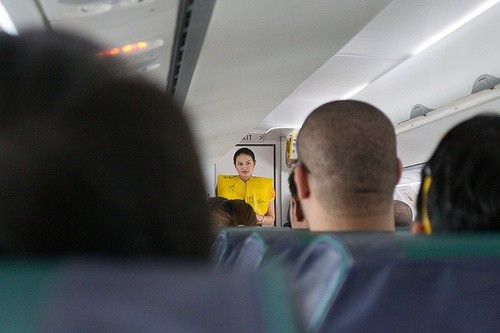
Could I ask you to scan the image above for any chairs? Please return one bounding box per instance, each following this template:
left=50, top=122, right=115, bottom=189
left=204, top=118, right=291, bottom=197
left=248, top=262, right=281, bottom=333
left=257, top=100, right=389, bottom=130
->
left=0, top=226, right=500, bottom=333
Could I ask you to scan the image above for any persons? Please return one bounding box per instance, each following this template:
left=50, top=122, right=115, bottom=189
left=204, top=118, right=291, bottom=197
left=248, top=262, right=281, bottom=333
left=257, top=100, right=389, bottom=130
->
left=1, top=29, right=214, bottom=271
left=287, top=171, right=308, bottom=230
left=393, top=200, right=414, bottom=227
left=414, top=112, right=500, bottom=236
left=216, top=147, right=275, bottom=228
left=293, top=100, right=403, bottom=233
left=208, top=197, right=256, bottom=228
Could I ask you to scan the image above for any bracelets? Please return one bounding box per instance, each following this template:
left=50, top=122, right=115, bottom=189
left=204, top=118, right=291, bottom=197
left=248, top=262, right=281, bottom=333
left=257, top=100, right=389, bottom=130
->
left=257, top=215, right=265, bottom=223
